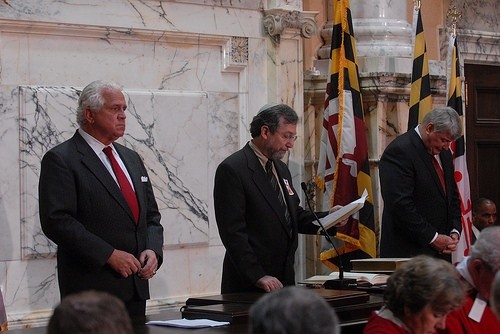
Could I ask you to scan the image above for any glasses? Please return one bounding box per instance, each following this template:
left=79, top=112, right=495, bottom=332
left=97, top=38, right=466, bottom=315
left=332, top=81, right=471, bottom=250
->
left=275, top=129, right=297, bottom=142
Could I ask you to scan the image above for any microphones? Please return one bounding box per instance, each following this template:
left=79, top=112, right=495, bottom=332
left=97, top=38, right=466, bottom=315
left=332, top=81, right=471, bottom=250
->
left=300, top=182, right=357, bottom=291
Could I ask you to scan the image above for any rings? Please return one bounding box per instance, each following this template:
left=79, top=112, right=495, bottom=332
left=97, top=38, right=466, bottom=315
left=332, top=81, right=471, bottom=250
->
left=153, top=270, right=156, bottom=275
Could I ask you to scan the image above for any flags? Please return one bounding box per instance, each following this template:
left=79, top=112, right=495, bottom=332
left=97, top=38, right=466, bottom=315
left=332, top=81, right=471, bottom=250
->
left=314, top=0, right=378, bottom=271
left=406, top=1, right=473, bottom=263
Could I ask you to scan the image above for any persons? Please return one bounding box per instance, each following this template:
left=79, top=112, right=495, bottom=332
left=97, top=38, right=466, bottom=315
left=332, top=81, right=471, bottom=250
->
left=243, top=285, right=342, bottom=334
left=46, top=292, right=135, bottom=334
left=379, top=106, right=462, bottom=264
left=362, top=197, right=500, bottom=334
left=39, top=79, right=165, bottom=317
left=213, top=102, right=348, bottom=295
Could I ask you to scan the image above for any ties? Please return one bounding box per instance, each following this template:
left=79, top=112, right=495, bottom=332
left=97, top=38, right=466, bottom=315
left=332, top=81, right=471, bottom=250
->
left=429, top=155, right=445, bottom=193
left=265, top=160, right=290, bottom=224
left=102, top=147, right=139, bottom=222
